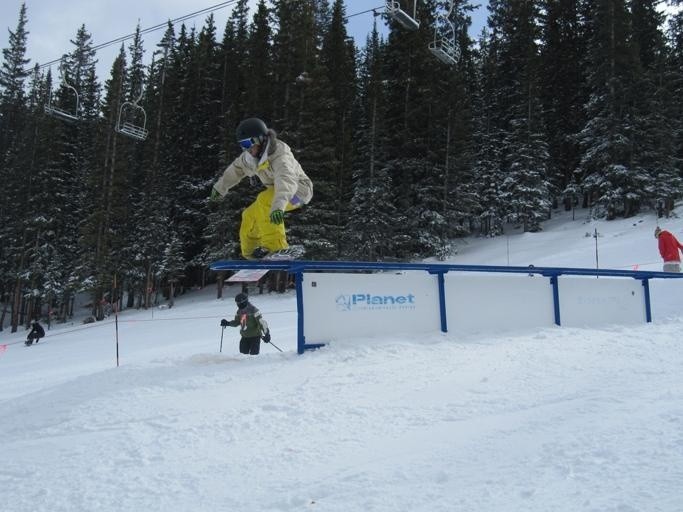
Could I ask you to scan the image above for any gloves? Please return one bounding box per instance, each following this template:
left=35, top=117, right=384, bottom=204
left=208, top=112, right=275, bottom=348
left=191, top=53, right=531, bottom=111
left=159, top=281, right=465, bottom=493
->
left=212, top=188, right=220, bottom=200
left=221, top=319, right=230, bottom=326
left=270, top=211, right=284, bottom=226
left=263, top=333, right=270, bottom=343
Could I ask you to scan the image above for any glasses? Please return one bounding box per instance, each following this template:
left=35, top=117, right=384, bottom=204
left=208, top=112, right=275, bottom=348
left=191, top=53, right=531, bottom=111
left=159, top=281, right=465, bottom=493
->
left=238, top=136, right=262, bottom=150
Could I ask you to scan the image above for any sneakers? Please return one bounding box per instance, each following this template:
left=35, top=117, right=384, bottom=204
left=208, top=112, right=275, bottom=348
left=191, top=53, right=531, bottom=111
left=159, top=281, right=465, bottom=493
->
left=252, top=245, right=273, bottom=258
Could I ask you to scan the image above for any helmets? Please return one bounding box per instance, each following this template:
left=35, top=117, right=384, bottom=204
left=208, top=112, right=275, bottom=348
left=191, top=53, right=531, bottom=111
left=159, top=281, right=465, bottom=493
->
left=235, top=293, right=248, bottom=309
left=236, top=118, right=268, bottom=139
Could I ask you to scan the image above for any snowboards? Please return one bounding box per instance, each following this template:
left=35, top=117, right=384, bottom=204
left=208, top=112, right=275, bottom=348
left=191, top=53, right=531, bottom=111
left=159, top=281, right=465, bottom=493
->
left=223, top=244, right=306, bottom=284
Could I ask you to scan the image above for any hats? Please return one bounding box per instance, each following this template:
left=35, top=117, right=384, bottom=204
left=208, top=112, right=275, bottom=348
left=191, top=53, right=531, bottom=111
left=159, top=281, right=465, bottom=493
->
left=655, top=226, right=661, bottom=236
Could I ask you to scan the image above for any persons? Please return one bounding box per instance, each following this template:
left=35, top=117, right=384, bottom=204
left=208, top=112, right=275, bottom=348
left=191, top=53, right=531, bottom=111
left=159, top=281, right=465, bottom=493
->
left=209, top=118, right=313, bottom=261
left=24, top=322, right=44, bottom=346
left=220, top=292, right=270, bottom=355
left=654, top=226, right=683, bottom=272
